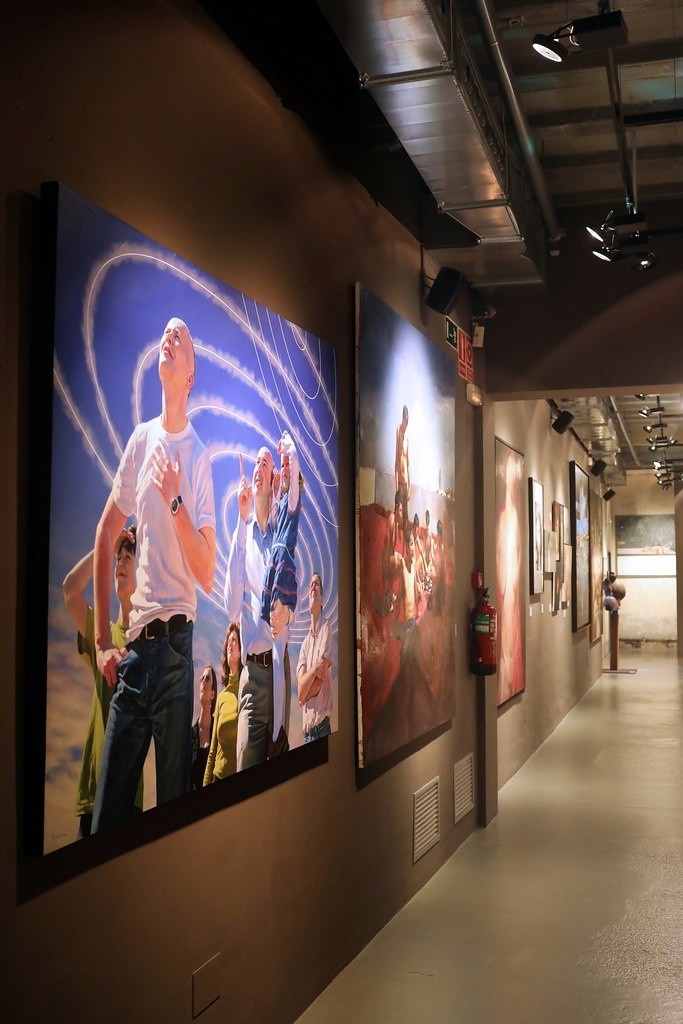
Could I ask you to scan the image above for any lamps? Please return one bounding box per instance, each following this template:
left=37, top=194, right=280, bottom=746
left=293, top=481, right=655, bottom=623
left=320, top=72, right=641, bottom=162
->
left=531, top=10, right=630, bottom=63
left=639, top=407, right=665, bottom=417
left=630, top=249, right=656, bottom=272
left=592, top=230, right=643, bottom=262
left=636, top=393, right=663, bottom=400
left=643, top=422, right=667, bottom=433
left=653, top=459, right=677, bottom=491
left=586, top=212, right=646, bottom=242
left=646, top=436, right=672, bottom=450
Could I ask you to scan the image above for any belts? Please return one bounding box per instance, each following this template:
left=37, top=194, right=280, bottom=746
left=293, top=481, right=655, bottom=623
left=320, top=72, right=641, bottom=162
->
left=140, top=615, right=186, bottom=640
left=305, top=716, right=327, bottom=736
left=247, top=650, right=272, bottom=668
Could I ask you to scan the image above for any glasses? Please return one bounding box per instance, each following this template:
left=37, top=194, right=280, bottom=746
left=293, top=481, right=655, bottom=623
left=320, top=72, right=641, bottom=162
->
left=200, top=676, right=211, bottom=683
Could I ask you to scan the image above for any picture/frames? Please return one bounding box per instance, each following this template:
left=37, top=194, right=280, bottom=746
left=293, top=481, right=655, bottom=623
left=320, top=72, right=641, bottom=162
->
left=570, top=460, right=593, bottom=633
left=529, top=477, right=545, bottom=595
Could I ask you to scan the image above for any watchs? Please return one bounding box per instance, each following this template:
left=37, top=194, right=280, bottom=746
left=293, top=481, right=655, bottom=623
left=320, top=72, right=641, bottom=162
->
left=168, top=495, right=182, bottom=515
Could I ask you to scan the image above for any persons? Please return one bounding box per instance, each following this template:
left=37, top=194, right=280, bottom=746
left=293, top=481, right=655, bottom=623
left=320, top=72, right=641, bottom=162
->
left=60, top=315, right=463, bottom=829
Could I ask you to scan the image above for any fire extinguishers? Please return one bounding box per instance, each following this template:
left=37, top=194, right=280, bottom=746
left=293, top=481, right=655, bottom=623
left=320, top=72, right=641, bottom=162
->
left=470, top=587, right=498, bottom=666
